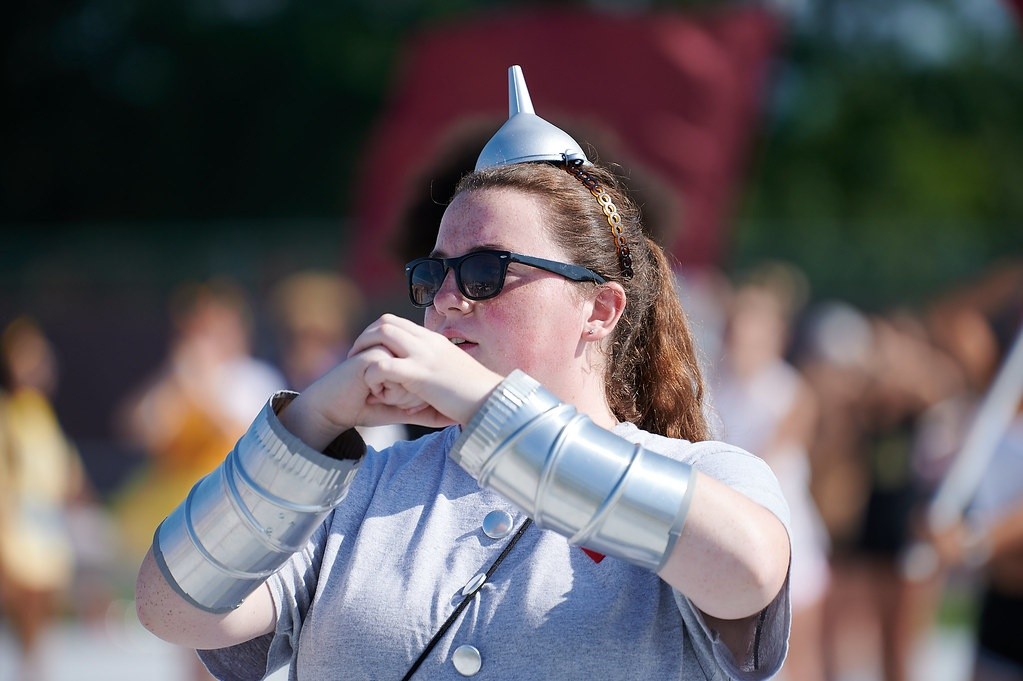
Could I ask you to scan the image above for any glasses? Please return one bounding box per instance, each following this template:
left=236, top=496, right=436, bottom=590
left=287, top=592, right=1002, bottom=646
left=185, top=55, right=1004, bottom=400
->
left=404, top=248, right=607, bottom=308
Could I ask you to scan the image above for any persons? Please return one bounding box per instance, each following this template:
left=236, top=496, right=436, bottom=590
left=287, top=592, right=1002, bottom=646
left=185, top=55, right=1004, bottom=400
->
left=0, top=242, right=1023, bottom=681
left=137, top=166, right=792, bottom=681
